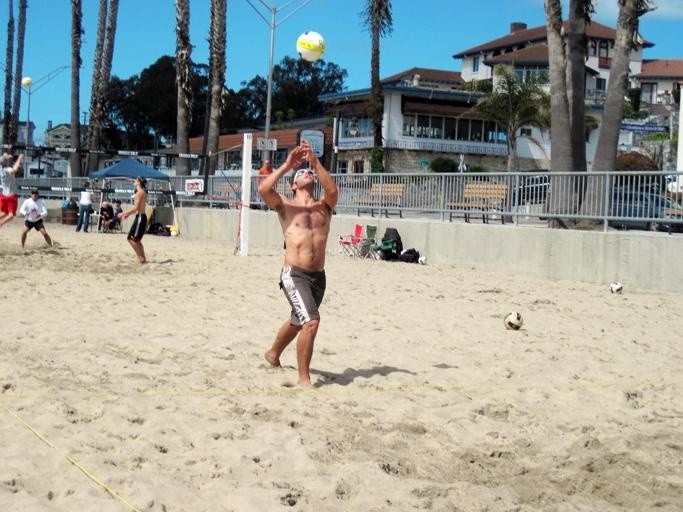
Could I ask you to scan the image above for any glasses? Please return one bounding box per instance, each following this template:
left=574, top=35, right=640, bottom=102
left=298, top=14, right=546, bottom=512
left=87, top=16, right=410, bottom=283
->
left=294, top=169, right=312, bottom=183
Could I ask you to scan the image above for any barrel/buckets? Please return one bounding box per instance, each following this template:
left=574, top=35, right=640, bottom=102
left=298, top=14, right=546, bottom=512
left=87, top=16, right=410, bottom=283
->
left=62, top=208, right=79, bottom=225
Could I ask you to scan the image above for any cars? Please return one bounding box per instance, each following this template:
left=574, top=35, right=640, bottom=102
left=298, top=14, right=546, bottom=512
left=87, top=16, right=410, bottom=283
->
left=610, top=191, right=683, bottom=232
left=505, top=166, right=551, bottom=202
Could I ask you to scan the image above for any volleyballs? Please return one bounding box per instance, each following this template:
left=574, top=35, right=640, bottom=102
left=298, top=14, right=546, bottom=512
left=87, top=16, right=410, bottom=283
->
left=297, top=31, right=325, bottom=60
left=419, top=256, right=426, bottom=264
left=504, top=311, right=522, bottom=329
left=610, top=281, right=621, bottom=291
left=22, top=77, right=31, bottom=86
left=171, top=230, right=177, bottom=236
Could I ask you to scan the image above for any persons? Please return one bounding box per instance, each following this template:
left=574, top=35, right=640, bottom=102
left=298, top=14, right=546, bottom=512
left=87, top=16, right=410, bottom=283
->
left=0, top=151, right=24, bottom=229
left=95, top=200, right=113, bottom=233
left=107, top=198, right=121, bottom=234
left=73, top=181, right=93, bottom=233
left=17, top=188, right=54, bottom=250
left=255, top=139, right=339, bottom=388
left=116, top=174, right=147, bottom=265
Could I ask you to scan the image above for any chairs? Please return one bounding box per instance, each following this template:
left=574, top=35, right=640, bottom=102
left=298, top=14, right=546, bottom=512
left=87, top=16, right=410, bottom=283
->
left=352, top=225, right=376, bottom=258
left=107, top=213, right=123, bottom=233
left=371, top=228, right=397, bottom=260
left=338, top=223, right=362, bottom=257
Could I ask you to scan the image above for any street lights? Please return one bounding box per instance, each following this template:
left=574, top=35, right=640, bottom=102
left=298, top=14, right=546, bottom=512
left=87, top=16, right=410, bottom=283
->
left=21, top=74, right=32, bottom=179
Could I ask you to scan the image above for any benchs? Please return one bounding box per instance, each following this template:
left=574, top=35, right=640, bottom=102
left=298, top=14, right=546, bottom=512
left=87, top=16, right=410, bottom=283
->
left=447, top=183, right=507, bottom=224
left=353, top=183, right=407, bottom=218
left=208, top=182, right=239, bottom=209
left=663, top=190, right=682, bottom=235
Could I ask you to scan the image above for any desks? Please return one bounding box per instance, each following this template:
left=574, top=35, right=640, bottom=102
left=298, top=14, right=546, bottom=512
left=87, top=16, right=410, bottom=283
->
left=75, top=213, right=105, bottom=235
